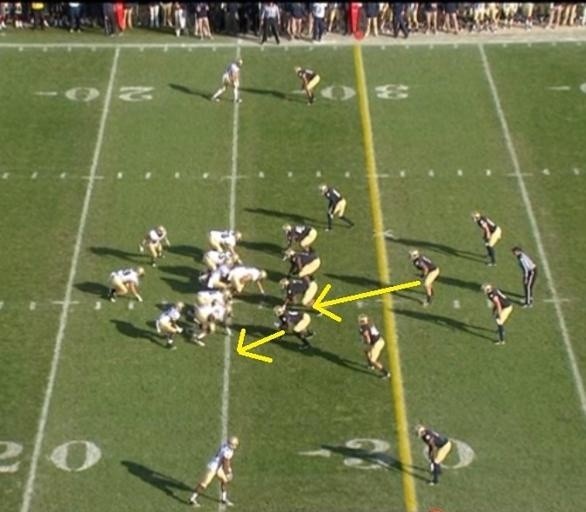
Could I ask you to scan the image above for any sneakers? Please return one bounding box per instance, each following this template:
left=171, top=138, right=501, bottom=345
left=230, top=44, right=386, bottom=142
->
left=164, top=338, right=206, bottom=347
left=379, top=372, right=391, bottom=380
left=427, top=480, right=440, bottom=486
left=521, top=297, right=534, bottom=309
left=423, top=300, right=432, bottom=306
left=187, top=498, right=200, bottom=507
left=486, top=254, right=496, bottom=267
left=299, top=331, right=316, bottom=350
left=493, top=340, right=505, bottom=345
left=220, top=499, right=234, bottom=506
left=109, top=288, right=117, bottom=303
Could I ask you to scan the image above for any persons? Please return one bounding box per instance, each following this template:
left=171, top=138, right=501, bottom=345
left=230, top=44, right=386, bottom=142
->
left=187, top=436, right=239, bottom=509
left=481, top=283, right=514, bottom=347
left=472, top=208, right=502, bottom=268
left=283, top=223, right=319, bottom=253
left=512, top=246, right=540, bottom=308
left=210, top=58, right=246, bottom=105
left=106, top=265, right=146, bottom=302
left=272, top=306, right=317, bottom=351
left=317, top=183, right=356, bottom=233
left=280, top=276, right=326, bottom=316
left=415, top=424, right=452, bottom=486
left=409, top=247, right=442, bottom=307
left=0, top=0, right=586, bottom=44
left=137, top=227, right=171, bottom=268
left=357, top=315, right=391, bottom=379
left=282, top=247, right=321, bottom=283
left=291, top=66, right=322, bottom=107
left=153, top=227, right=268, bottom=350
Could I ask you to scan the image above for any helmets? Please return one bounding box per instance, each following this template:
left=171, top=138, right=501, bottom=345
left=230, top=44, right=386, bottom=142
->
left=470, top=211, right=481, bottom=224
left=224, top=289, right=234, bottom=317
left=258, top=270, right=267, bottom=280
left=412, top=424, right=427, bottom=439
left=135, top=266, right=145, bottom=276
left=481, top=282, right=493, bottom=295
left=231, top=231, right=242, bottom=263
left=272, top=224, right=296, bottom=317
left=174, top=301, right=186, bottom=311
left=227, top=436, right=239, bottom=448
left=156, top=225, right=165, bottom=235
left=356, top=314, right=369, bottom=326
left=317, top=182, right=329, bottom=196
left=408, top=249, right=419, bottom=261
